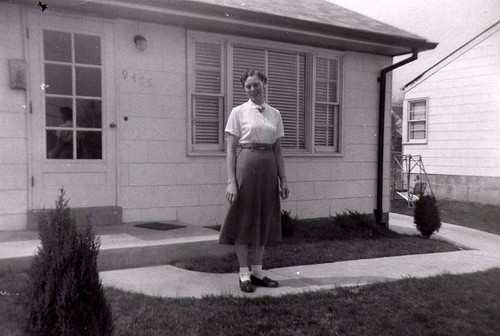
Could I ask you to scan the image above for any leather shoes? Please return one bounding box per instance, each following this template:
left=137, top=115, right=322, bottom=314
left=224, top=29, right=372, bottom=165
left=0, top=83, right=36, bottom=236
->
left=251, top=273, right=278, bottom=288
left=239, top=276, right=253, bottom=292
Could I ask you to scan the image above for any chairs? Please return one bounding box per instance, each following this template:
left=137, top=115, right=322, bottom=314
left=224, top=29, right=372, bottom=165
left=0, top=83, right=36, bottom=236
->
left=396, top=182, right=427, bottom=208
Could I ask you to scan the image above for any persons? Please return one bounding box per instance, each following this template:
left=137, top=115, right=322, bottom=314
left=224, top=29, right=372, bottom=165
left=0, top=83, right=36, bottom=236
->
left=47, top=107, right=89, bottom=159
left=224, top=71, right=288, bottom=291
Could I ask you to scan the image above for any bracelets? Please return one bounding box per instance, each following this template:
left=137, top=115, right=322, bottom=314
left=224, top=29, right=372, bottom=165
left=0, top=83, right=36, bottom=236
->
left=280, top=176, right=286, bottom=180
